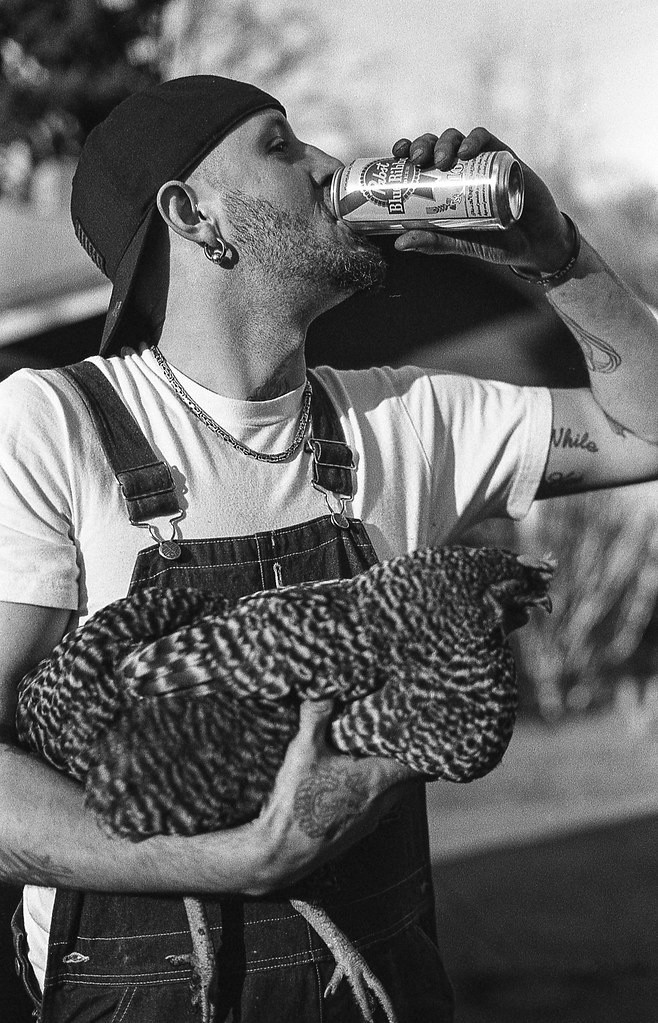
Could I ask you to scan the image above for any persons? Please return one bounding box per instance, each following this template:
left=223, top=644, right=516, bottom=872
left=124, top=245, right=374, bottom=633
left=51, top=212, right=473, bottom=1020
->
left=0, top=74, right=658, bottom=1022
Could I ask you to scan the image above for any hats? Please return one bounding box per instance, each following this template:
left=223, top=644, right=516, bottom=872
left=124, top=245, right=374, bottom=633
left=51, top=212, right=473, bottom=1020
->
left=70, top=74, right=288, bottom=360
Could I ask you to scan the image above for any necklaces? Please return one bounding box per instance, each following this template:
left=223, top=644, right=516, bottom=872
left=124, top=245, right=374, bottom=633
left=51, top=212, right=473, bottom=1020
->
left=151, top=345, right=313, bottom=462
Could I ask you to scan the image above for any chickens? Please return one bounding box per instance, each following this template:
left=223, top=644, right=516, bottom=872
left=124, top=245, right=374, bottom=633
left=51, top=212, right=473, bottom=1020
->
left=11, top=542, right=555, bottom=1023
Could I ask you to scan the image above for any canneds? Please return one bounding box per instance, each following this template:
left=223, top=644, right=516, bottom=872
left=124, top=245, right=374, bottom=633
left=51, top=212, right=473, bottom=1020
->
left=330, top=149, right=524, bottom=236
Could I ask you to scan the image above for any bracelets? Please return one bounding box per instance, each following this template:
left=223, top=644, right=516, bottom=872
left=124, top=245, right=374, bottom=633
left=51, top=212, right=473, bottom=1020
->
left=506, top=211, right=580, bottom=284
